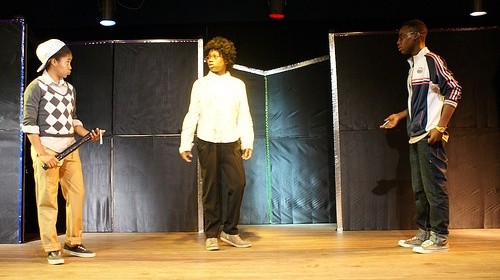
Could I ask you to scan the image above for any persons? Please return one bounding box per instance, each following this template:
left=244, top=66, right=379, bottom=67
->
left=22, top=39, right=105, bottom=265
left=179, top=37, right=254, bottom=251
left=380, top=19, right=462, bottom=254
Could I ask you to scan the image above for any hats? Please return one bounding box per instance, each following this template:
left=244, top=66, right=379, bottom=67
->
left=35, top=38, right=66, bottom=73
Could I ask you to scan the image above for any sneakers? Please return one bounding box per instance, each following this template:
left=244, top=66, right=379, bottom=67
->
left=47, top=250, right=64, bottom=265
left=220, top=230, right=252, bottom=248
left=413, top=240, right=449, bottom=253
left=205, top=238, right=219, bottom=251
left=398, top=236, right=423, bottom=248
left=63, top=242, right=96, bottom=258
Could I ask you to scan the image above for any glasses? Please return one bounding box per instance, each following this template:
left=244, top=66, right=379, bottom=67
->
left=398, top=32, right=417, bottom=41
left=206, top=55, right=222, bottom=60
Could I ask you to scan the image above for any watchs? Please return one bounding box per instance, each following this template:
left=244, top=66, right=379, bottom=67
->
left=436, top=126, right=445, bottom=132
